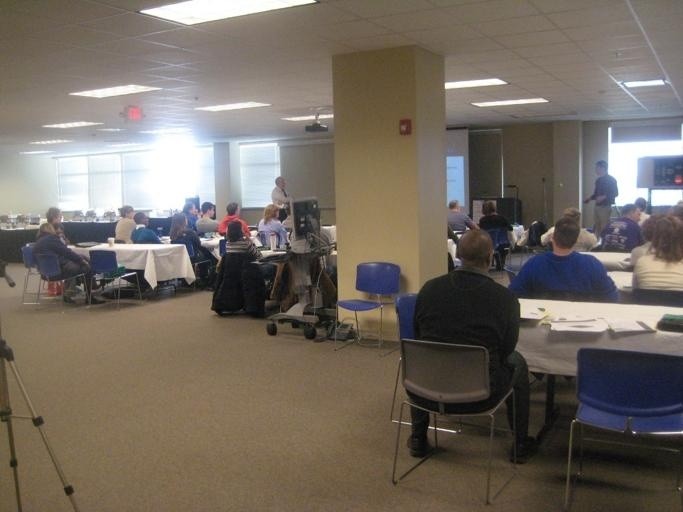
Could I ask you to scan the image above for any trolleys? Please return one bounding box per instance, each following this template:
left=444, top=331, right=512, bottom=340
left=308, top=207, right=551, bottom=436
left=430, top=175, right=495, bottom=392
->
left=254, top=244, right=337, bottom=339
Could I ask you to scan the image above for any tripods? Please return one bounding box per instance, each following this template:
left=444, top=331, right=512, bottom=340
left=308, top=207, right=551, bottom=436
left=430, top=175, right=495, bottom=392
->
left=0, top=255, right=81, bottom=512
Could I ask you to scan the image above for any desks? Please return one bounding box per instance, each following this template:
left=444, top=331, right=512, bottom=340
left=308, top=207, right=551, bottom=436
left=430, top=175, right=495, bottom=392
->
left=147, top=210, right=175, bottom=238
left=514, top=298, right=683, bottom=444
left=0, top=222, right=42, bottom=286
left=161, top=235, right=225, bottom=272
left=259, top=249, right=288, bottom=300
left=577, top=251, right=633, bottom=270
left=608, top=271, right=633, bottom=304
left=61, top=218, right=118, bottom=245
left=67, top=243, right=196, bottom=294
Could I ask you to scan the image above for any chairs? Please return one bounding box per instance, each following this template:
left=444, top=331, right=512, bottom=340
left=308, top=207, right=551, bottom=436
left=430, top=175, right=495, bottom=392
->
left=35, top=254, right=88, bottom=304
left=25, top=243, right=45, bottom=288
left=258, top=231, right=280, bottom=248
left=219, top=240, right=226, bottom=256
left=20, top=246, right=38, bottom=303
left=488, top=228, right=512, bottom=269
left=389, top=293, right=463, bottom=436
left=170, top=238, right=212, bottom=289
left=389, top=338, right=517, bottom=506
left=210, top=252, right=266, bottom=317
left=89, top=250, right=142, bottom=310
left=334, top=261, right=400, bottom=357
left=564, top=347, right=683, bottom=512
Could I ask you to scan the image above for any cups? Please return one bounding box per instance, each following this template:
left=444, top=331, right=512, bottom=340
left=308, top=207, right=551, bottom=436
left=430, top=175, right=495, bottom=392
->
left=108, top=238, right=114, bottom=247
left=157, top=227, right=163, bottom=237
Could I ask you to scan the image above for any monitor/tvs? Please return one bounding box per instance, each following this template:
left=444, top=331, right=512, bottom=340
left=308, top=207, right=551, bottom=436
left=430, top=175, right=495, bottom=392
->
left=289, top=195, right=330, bottom=253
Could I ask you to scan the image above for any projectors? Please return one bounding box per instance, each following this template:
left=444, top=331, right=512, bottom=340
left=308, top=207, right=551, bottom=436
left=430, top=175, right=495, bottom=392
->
left=305, top=120, right=328, bottom=133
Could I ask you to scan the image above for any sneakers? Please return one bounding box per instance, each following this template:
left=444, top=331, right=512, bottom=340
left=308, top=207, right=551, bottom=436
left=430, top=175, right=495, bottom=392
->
left=85, top=297, right=106, bottom=305
left=63, top=295, right=75, bottom=304
left=406, top=435, right=430, bottom=457
left=511, top=436, right=537, bottom=463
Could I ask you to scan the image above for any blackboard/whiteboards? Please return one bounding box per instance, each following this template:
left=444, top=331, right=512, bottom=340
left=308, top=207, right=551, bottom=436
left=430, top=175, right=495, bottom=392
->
left=655, top=156, right=683, bottom=190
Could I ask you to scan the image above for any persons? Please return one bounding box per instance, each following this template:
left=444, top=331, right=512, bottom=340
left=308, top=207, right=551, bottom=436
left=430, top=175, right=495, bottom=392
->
left=131, top=213, right=163, bottom=244
left=45, top=208, right=70, bottom=245
left=195, top=202, right=221, bottom=236
left=601, top=204, right=645, bottom=253
left=272, top=176, right=295, bottom=222
left=583, top=160, right=618, bottom=234
left=116, top=206, right=137, bottom=244
left=631, top=216, right=660, bottom=268
left=170, top=214, right=218, bottom=280
left=33, top=223, right=107, bottom=304
left=632, top=217, right=683, bottom=290
left=447, top=224, right=459, bottom=244
left=218, top=202, right=251, bottom=237
left=540, top=208, right=597, bottom=253
left=181, top=202, right=199, bottom=232
left=226, top=220, right=264, bottom=260
left=508, top=218, right=620, bottom=303
left=407, top=228, right=539, bottom=463
left=635, top=198, right=651, bottom=226
left=447, top=201, right=481, bottom=231
left=258, top=205, right=288, bottom=248
left=479, top=202, right=513, bottom=271
